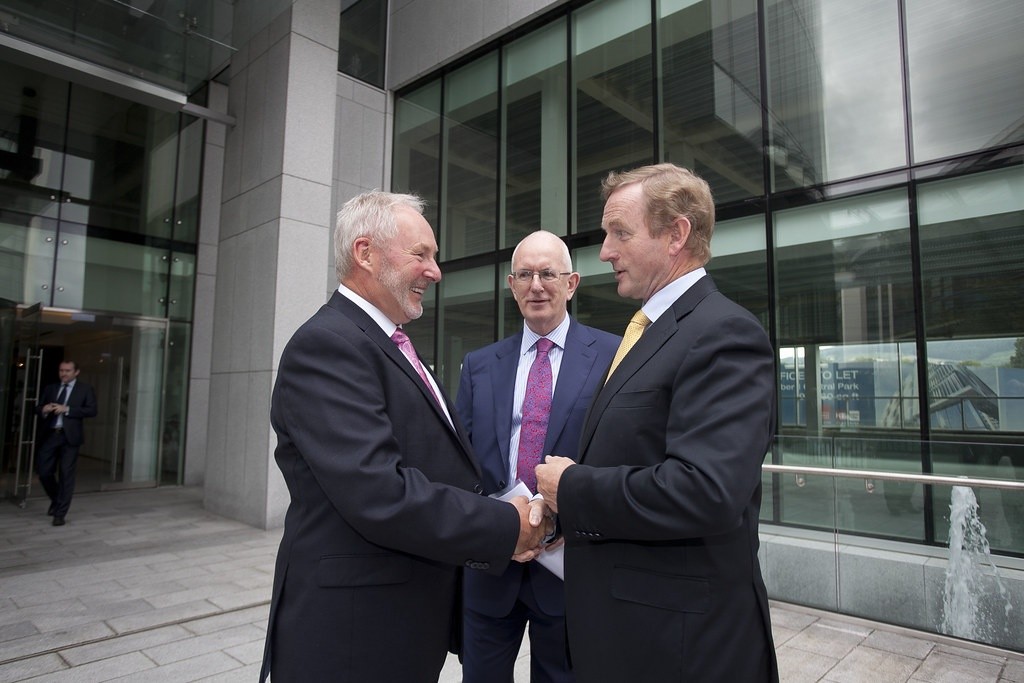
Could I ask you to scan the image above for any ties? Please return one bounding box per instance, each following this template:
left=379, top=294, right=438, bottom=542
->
left=51, top=384, right=70, bottom=427
left=603, top=309, right=651, bottom=388
left=516, top=338, right=555, bottom=496
left=390, top=327, right=441, bottom=407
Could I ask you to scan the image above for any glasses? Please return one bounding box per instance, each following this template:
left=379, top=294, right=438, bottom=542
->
left=511, top=269, right=571, bottom=283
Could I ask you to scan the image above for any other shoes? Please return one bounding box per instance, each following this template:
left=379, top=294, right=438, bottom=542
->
left=51, top=516, right=66, bottom=526
left=47, top=500, right=56, bottom=516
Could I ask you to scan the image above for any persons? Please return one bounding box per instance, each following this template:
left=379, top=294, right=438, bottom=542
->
left=34, top=358, right=96, bottom=526
left=259, top=189, right=556, bottom=683
left=877, top=436, right=1024, bottom=531
left=510, top=162, right=780, bottom=683
left=454, top=230, right=624, bottom=683
